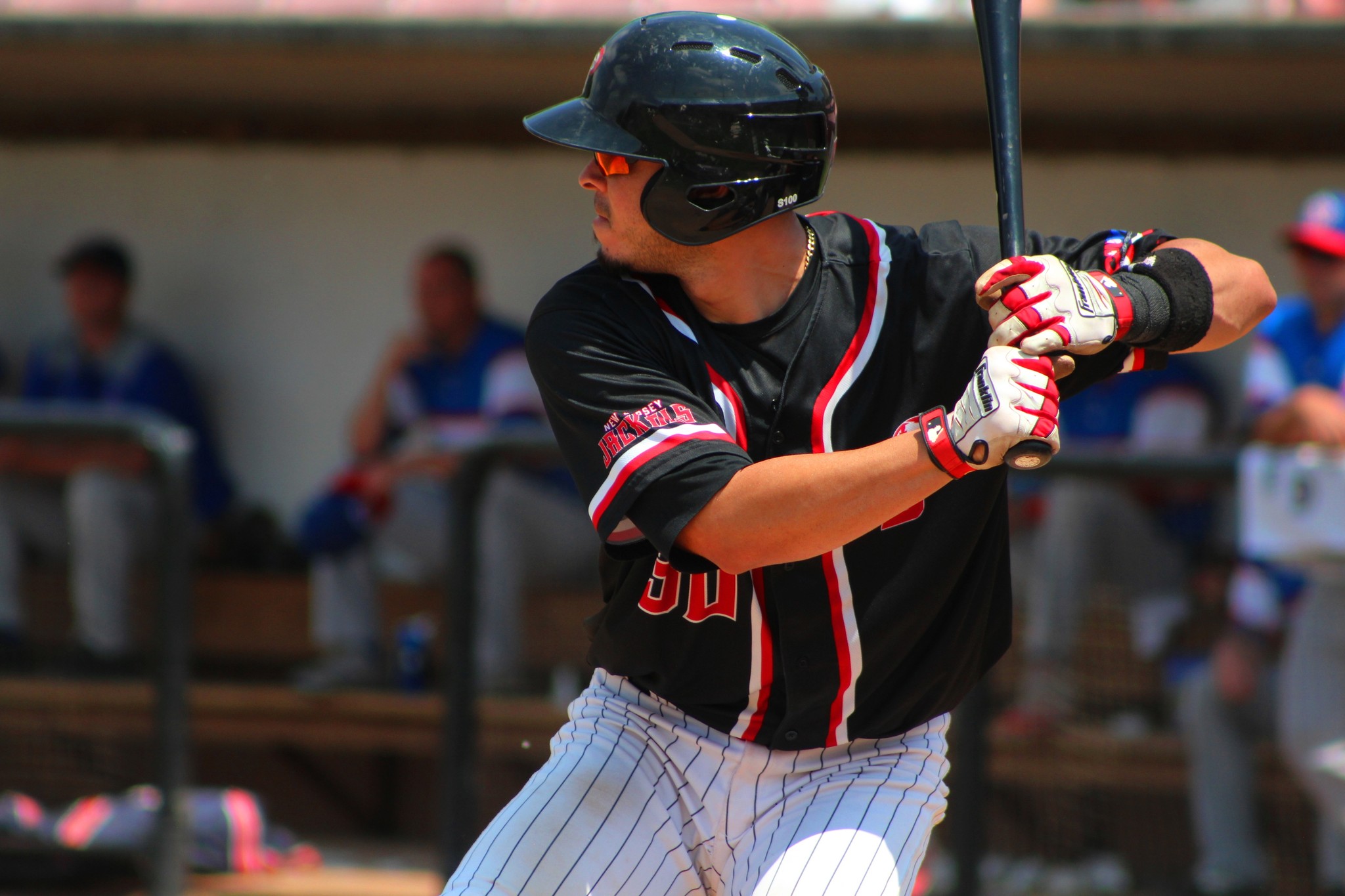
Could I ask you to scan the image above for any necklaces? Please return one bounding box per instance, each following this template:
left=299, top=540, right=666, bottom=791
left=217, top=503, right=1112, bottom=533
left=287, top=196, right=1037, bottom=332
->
left=805, top=225, right=815, bottom=270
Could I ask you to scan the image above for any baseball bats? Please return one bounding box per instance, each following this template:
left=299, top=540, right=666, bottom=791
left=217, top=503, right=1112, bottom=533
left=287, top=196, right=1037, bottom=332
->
left=968, top=1, right=1057, bottom=474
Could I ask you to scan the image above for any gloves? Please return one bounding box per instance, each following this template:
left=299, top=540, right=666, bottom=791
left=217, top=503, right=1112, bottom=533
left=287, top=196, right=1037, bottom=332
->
left=919, top=346, right=1074, bottom=479
left=975, top=254, right=1134, bottom=354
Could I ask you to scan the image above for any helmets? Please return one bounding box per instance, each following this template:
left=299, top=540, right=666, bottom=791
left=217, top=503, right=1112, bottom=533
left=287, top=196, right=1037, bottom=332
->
left=522, top=11, right=838, bottom=247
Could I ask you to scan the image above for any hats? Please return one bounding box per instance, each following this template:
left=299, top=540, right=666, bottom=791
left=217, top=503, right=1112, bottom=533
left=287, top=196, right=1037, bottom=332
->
left=1285, top=189, right=1345, bottom=258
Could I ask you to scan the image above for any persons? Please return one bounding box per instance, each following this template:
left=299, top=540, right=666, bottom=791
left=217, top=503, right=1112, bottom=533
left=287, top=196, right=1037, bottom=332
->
left=1, top=225, right=227, bottom=647
left=1216, top=196, right=1345, bottom=896
left=1017, top=362, right=1261, bottom=896
left=287, top=238, right=541, bottom=692
left=440, top=11, right=1277, bottom=896
left=479, top=478, right=599, bottom=691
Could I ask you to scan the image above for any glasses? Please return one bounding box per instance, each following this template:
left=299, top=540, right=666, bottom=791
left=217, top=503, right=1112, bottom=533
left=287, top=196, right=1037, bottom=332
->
left=593, top=150, right=641, bottom=175
